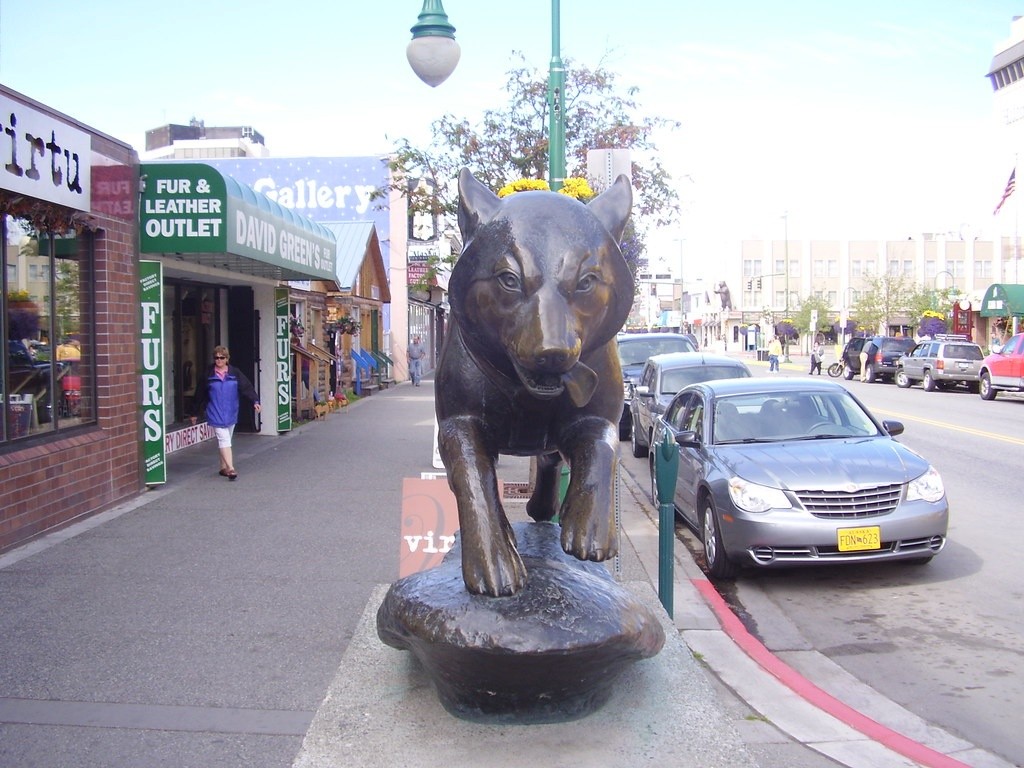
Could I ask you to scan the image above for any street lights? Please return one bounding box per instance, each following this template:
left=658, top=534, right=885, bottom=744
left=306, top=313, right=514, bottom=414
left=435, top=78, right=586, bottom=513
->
left=407, top=0, right=566, bottom=194
left=674, top=238, right=687, bottom=335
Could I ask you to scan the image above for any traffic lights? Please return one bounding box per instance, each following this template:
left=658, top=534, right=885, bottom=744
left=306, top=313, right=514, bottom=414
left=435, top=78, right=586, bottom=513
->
left=748, top=281, right=752, bottom=290
left=757, top=279, right=762, bottom=289
left=651, top=284, right=656, bottom=295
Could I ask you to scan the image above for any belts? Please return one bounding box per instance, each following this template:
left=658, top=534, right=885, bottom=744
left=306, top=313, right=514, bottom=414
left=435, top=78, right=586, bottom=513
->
left=410, top=356, right=418, bottom=360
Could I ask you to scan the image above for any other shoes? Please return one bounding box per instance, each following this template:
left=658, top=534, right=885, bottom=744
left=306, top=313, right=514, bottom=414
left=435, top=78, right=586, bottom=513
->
left=412, top=380, right=415, bottom=385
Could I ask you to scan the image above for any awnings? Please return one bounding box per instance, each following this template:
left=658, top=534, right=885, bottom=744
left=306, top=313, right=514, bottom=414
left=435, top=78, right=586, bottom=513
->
left=140, top=161, right=336, bottom=281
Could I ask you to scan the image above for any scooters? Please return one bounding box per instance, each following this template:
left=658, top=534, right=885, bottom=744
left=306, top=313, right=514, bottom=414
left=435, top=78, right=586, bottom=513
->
left=827, top=355, right=846, bottom=377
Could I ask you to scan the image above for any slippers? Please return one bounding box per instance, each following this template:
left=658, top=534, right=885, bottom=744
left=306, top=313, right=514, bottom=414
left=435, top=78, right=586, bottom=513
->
left=219, top=468, right=228, bottom=475
left=227, top=470, right=237, bottom=478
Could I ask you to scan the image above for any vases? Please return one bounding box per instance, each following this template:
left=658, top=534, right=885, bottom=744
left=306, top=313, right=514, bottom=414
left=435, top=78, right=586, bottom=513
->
left=346, top=326, right=356, bottom=335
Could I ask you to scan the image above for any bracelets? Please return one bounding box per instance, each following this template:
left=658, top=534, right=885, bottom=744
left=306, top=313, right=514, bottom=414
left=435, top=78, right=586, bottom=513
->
left=785, top=400, right=789, bottom=408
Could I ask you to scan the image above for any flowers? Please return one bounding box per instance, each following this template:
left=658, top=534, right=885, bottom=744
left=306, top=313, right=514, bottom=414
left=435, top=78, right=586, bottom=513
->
left=290, top=316, right=305, bottom=338
left=333, top=316, right=362, bottom=337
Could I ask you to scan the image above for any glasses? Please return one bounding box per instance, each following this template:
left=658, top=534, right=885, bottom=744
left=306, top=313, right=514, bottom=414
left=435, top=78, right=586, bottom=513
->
left=214, top=356, right=227, bottom=359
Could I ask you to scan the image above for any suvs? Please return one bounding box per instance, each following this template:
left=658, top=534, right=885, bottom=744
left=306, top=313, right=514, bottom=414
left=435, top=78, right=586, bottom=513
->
left=894, top=333, right=986, bottom=394
left=861, top=333, right=919, bottom=384
left=842, top=335, right=866, bottom=379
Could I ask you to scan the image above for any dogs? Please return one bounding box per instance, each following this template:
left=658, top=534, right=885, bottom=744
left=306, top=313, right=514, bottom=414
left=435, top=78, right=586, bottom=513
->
left=434, top=166, right=635, bottom=599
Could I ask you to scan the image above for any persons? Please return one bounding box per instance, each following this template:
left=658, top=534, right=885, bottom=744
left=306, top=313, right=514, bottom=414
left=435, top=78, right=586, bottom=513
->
left=190, top=346, right=262, bottom=480
left=990, top=332, right=1000, bottom=355
left=809, top=341, right=824, bottom=375
left=767, top=335, right=783, bottom=373
left=760, top=399, right=801, bottom=435
left=406, top=337, right=425, bottom=386
left=859, top=341, right=878, bottom=383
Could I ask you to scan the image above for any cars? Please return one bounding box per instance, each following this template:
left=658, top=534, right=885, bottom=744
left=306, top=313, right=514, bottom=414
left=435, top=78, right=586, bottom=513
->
left=615, top=332, right=703, bottom=442
left=978, top=331, right=1024, bottom=400
left=629, top=352, right=763, bottom=459
left=4, top=339, right=82, bottom=425
left=648, top=376, right=949, bottom=583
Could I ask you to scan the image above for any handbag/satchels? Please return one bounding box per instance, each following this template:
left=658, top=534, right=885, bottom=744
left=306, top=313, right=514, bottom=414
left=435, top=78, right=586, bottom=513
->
left=778, top=355, right=786, bottom=363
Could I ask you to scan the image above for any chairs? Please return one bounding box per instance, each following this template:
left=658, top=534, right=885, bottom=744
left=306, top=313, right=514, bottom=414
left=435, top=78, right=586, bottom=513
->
left=713, top=401, right=749, bottom=442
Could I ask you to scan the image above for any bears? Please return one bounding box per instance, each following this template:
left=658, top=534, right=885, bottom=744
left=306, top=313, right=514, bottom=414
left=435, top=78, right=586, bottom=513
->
left=713, top=281, right=733, bottom=312
